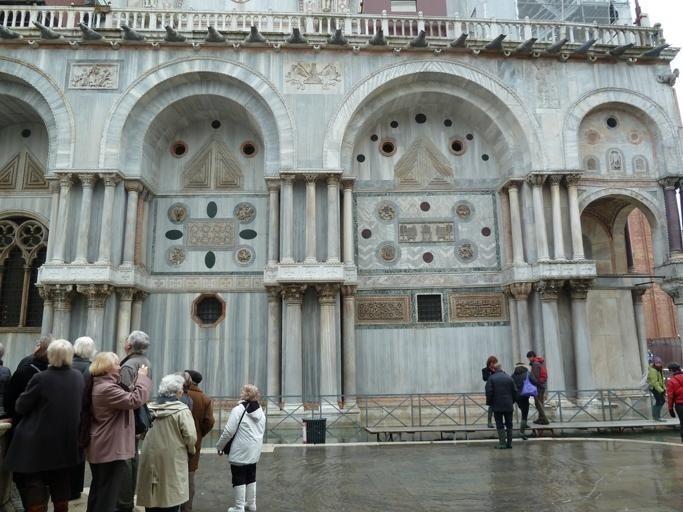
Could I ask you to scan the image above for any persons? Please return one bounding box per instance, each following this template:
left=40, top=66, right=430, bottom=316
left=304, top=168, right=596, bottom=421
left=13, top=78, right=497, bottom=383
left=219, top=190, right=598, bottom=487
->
left=486, top=363, right=518, bottom=448
left=647, top=357, right=666, bottom=422
left=667, top=362, right=683, bottom=443
left=527, top=351, right=549, bottom=424
left=483, top=356, right=498, bottom=428
left=218, top=384, right=266, bottom=512
left=1, top=330, right=216, bottom=507
left=511, top=363, right=537, bottom=440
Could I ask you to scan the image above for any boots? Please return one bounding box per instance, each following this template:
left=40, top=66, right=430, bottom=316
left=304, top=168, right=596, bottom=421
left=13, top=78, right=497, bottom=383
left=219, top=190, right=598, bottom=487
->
left=227, top=482, right=258, bottom=512
left=518, top=418, right=530, bottom=441
left=494, top=424, right=513, bottom=450
left=486, top=410, right=495, bottom=429
left=651, top=404, right=668, bottom=424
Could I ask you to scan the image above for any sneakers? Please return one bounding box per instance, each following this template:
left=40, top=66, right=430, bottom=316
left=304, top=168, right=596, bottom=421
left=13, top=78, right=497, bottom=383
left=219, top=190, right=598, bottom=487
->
left=533, top=416, right=549, bottom=425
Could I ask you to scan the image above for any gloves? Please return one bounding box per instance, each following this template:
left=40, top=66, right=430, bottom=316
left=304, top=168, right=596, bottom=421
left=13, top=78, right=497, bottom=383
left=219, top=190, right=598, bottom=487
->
left=669, top=409, right=676, bottom=418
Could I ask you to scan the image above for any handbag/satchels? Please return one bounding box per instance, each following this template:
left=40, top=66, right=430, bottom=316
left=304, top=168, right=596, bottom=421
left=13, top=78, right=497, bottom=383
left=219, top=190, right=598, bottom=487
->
left=519, top=371, right=537, bottom=397
left=221, top=434, right=235, bottom=454
left=134, top=401, right=154, bottom=435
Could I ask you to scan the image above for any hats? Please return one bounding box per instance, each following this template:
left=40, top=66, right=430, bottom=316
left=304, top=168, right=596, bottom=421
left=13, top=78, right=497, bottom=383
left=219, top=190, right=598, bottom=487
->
left=652, top=354, right=665, bottom=365
